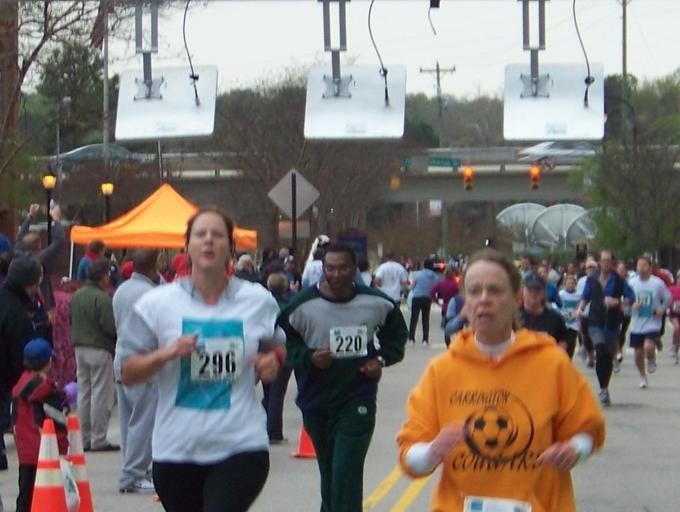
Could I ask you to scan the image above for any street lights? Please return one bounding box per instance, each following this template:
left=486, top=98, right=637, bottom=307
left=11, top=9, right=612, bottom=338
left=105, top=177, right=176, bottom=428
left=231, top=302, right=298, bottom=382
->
left=101, top=178, right=114, bottom=223
left=42, top=164, right=59, bottom=245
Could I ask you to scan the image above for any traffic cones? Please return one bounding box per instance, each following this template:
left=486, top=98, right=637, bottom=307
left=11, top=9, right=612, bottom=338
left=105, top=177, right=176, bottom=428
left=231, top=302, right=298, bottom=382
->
left=62, top=414, right=94, bottom=511
left=28, top=417, right=71, bottom=511
left=289, top=422, right=319, bottom=459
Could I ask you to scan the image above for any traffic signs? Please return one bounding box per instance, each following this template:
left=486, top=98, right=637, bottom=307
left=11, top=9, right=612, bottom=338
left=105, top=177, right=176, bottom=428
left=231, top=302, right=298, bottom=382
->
left=405, top=156, right=461, bottom=167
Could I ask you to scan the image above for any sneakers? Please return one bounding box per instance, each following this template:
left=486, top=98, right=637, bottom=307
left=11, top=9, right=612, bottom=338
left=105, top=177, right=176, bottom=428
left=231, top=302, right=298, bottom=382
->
left=639, top=376, right=649, bottom=389
left=670, top=344, right=679, bottom=364
left=119, top=480, right=154, bottom=495
left=599, top=389, right=609, bottom=405
left=647, top=359, right=657, bottom=374
left=586, top=357, right=595, bottom=372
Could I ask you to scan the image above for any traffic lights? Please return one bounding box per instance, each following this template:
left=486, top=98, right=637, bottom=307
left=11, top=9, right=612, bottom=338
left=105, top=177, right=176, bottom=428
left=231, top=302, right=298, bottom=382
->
left=530, top=166, right=540, bottom=190
left=465, top=167, right=473, bottom=191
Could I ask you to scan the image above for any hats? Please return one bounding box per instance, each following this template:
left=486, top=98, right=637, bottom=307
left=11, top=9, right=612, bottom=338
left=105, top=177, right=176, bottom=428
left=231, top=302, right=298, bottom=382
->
left=23, top=338, right=57, bottom=364
left=7, top=251, right=43, bottom=288
left=523, top=272, right=546, bottom=289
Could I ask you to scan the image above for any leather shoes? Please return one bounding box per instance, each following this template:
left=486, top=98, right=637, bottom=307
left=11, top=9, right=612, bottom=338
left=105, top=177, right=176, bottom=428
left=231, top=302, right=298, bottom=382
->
left=89, top=440, right=121, bottom=453
